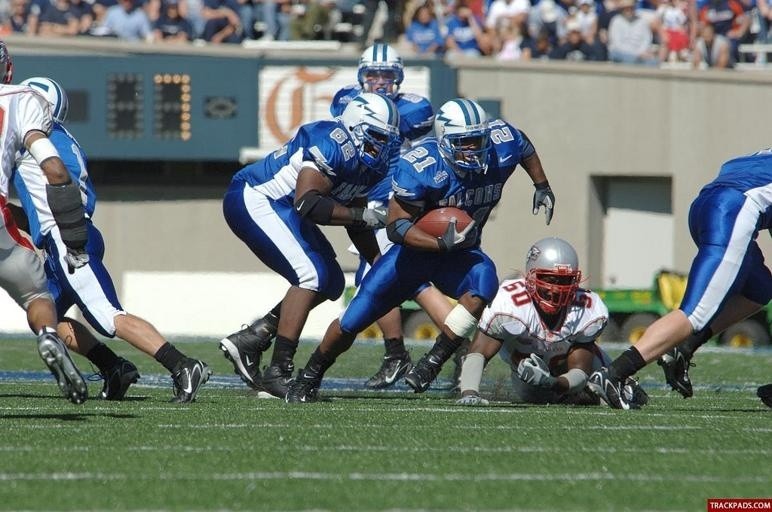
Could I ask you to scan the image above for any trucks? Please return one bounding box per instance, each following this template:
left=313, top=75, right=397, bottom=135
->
left=341, top=268, right=772, bottom=350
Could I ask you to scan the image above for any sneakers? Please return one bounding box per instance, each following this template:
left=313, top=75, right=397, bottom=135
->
left=35, top=324, right=88, bottom=406
left=87, top=358, right=140, bottom=401
left=284, top=362, right=325, bottom=404
left=364, top=351, right=412, bottom=389
left=586, top=367, right=630, bottom=409
left=405, top=353, right=442, bottom=395
left=621, top=375, right=649, bottom=407
left=170, top=357, right=213, bottom=404
left=657, top=346, right=697, bottom=399
left=219, top=324, right=272, bottom=392
left=262, top=361, right=320, bottom=403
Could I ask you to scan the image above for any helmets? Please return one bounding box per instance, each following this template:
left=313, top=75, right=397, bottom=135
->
left=524, top=235, right=580, bottom=315
left=341, top=91, right=400, bottom=173
left=0, top=41, right=13, bottom=84
left=357, top=42, right=405, bottom=100
left=20, top=77, right=69, bottom=125
left=433, top=97, right=489, bottom=170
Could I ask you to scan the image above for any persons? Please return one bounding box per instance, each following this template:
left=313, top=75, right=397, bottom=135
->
left=581, top=144, right=771, bottom=412
left=20, top=76, right=214, bottom=404
left=1, top=0, right=771, bottom=70
left=753, top=378, right=771, bottom=413
left=217, top=89, right=405, bottom=401
left=327, top=42, right=473, bottom=398
left=453, top=233, right=650, bottom=411
left=280, top=95, right=559, bottom=405
left=0, top=39, right=91, bottom=407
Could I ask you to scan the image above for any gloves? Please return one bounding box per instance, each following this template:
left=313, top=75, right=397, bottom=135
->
left=455, top=394, right=491, bottom=408
left=436, top=216, right=478, bottom=254
left=532, top=181, right=556, bottom=224
left=64, top=247, right=90, bottom=274
left=515, top=353, right=554, bottom=388
left=361, top=207, right=388, bottom=231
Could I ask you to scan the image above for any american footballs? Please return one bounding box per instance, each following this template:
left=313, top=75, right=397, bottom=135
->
left=414, top=208, right=473, bottom=235
